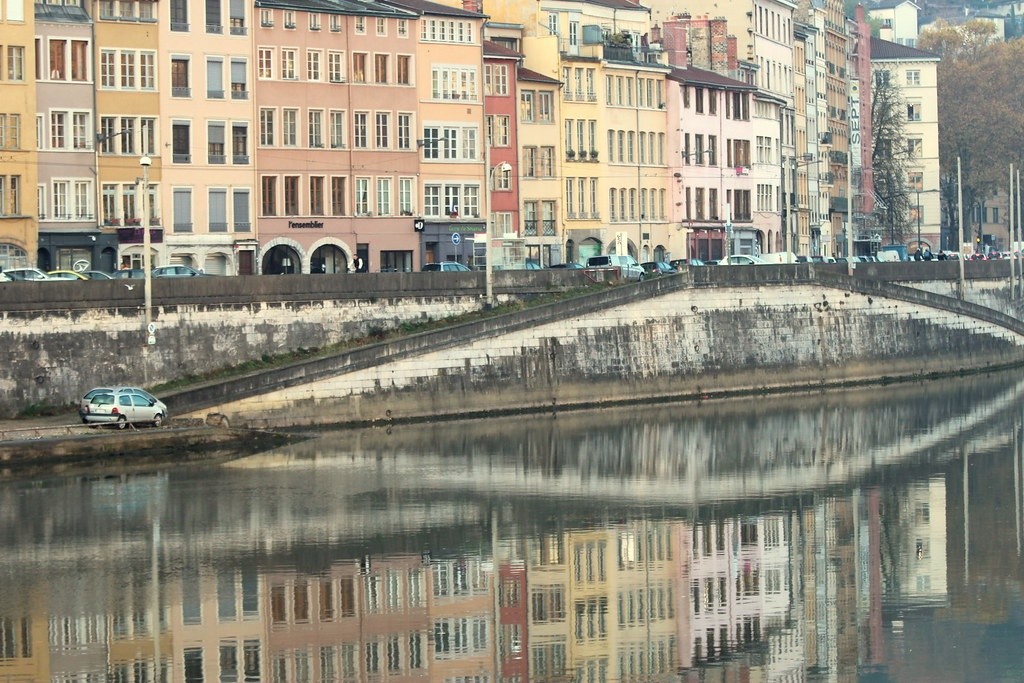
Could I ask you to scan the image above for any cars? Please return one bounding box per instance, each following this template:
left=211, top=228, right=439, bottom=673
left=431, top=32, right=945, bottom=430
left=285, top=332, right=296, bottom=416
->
left=152, top=263, right=202, bottom=277
left=797, top=255, right=881, bottom=263
left=547, top=263, right=585, bottom=270
left=3, top=267, right=69, bottom=281
left=909, top=250, right=1024, bottom=261
left=640, top=262, right=677, bottom=277
left=81, top=271, right=117, bottom=280
left=83, top=392, right=166, bottom=430
left=718, top=254, right=767, bottom=265
left=111, top=268, right=158, bottom=279
left=47, top=270, right=89, bottom=280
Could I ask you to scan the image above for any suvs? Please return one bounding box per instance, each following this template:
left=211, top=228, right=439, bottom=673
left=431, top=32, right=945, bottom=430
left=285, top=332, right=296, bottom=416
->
left=78, top=386, right=168, bottom=424
left=421, top=261, right=471, bottom=271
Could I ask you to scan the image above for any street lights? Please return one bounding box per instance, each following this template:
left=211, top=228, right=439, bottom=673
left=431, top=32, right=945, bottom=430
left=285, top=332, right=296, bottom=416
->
left=134, top=153, right=152, bottom=326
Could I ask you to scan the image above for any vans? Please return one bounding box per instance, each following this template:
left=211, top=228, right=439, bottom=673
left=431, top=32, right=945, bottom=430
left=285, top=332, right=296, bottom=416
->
left=876, top=250, right=901, bottom=263
left=586, top=254, right=645, bottom=283
left=759, top=251, right=801, bottom=264
left=669, top=259, right=707, bottom=271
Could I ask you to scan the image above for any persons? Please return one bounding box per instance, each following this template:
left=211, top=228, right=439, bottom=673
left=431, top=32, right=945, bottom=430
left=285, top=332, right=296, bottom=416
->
left=118, top=260, right=130, bottom=270
left=353, top=254, right=367, bottom=273
left=642, top=33, right=651, bottom=63
left=914, top=247, right=947, bottom=261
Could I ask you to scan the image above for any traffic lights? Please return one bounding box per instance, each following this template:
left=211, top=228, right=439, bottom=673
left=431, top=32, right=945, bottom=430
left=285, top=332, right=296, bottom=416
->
left=977, top=235, right=982, bottom=244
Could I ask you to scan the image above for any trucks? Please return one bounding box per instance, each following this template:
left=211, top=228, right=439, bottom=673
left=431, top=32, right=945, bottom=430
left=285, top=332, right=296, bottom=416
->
left=882, top=244, right=908, bottom=262
